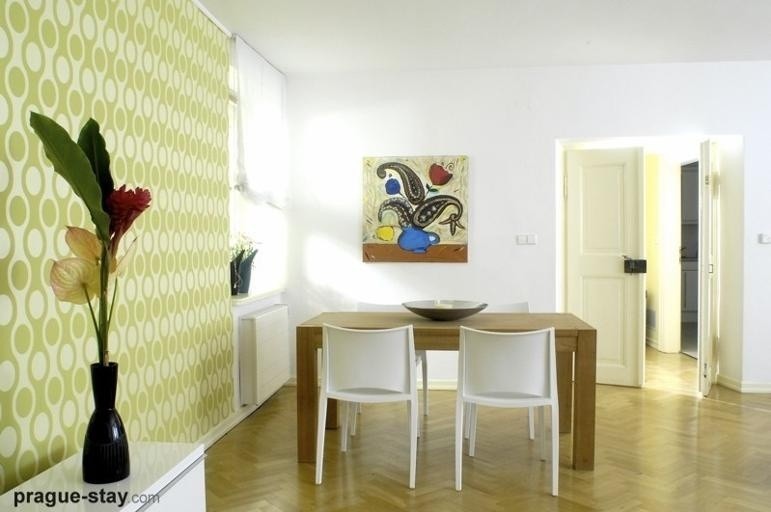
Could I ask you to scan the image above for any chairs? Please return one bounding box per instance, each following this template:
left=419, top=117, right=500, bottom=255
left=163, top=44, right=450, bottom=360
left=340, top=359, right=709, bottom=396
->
left=312, top=320, right=565, bottom=498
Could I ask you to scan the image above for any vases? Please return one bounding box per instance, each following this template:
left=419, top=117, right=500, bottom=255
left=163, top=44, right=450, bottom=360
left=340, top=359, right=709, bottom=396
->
left=81, top=359, right=131, bottom=485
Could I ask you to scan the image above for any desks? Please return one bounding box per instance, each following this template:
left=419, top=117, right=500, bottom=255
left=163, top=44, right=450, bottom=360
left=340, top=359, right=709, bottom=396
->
left=293, top=300, right=597, bottom=476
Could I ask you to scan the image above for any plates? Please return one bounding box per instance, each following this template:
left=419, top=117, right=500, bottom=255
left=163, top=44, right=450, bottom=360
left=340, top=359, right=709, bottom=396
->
left=401, top=300, right=488, bottom=321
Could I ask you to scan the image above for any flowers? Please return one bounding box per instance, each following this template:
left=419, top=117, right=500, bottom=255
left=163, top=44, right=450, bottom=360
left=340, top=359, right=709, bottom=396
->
left=31, top=108, right=154, bottom=367
